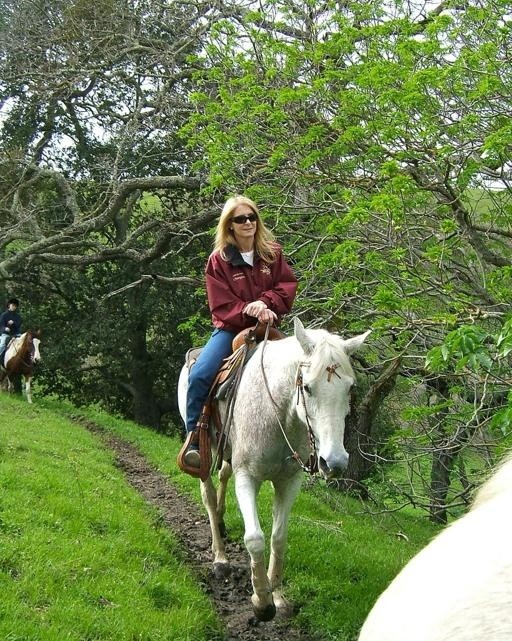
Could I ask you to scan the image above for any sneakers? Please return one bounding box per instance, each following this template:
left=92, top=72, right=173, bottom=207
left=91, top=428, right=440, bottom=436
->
left=183, top=445, right=201, bottom=467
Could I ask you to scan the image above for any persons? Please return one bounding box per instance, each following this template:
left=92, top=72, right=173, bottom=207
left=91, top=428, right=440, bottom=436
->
left=184, top=195, right=299, bottom=468
left=0, top=298, right=23, bottom=356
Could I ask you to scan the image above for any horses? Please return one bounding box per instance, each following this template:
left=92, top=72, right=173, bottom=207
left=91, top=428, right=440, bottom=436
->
left=0, top=326, right=43, bottom=406
left=177, top=315, right=374, bottom=621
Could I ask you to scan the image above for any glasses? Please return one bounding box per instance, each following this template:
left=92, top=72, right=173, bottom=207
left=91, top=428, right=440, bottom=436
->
left=230, top=213, right=257, bottom=224
